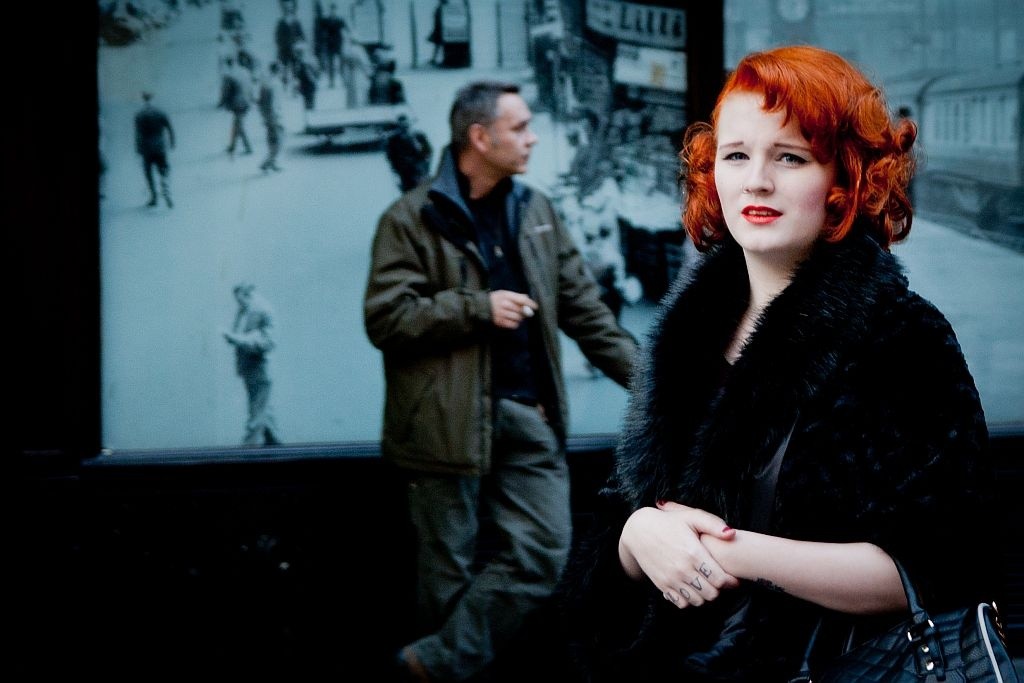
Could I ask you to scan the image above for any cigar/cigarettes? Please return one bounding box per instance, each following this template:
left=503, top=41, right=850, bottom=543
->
left=522, top=306, right=535, bottom=316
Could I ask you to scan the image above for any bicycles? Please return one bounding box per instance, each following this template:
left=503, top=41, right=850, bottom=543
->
left=350, top=40, right=405, bottom=106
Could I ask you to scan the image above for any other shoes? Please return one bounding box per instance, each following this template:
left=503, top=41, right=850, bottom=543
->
left=398, top=645, right=427, bottom=682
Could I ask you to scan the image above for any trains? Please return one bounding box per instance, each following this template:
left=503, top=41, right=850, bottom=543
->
left=886, top=58, right=1023, bottom=258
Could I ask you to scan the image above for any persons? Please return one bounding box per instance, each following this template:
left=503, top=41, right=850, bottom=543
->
left=97, top=0, right=643, bottom=372
left=219, top=281, right=282, bottom=446
left=362, top=81, right=643, bottom=683
left=600, top=47, right=992, bottom=683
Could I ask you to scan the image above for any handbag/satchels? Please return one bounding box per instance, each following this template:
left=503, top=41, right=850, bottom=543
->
left=790, top=557, right=1024, bottom=683
